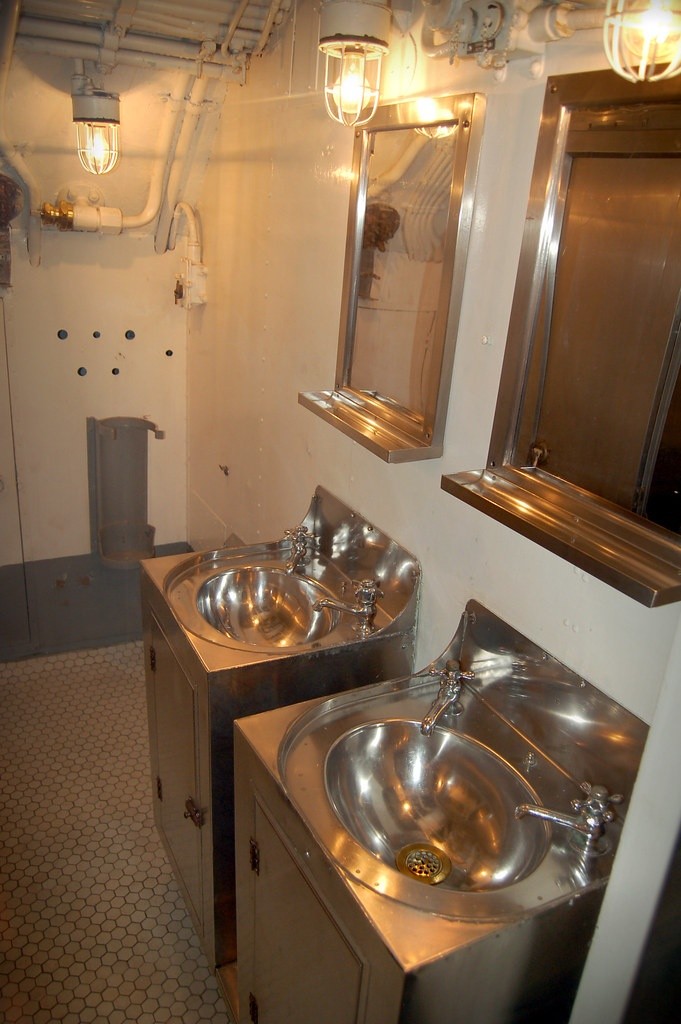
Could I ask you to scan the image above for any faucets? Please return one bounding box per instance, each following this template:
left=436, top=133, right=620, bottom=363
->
left=312, top=579, right=384, bottom=636
left=284, top=526, right=314, bottom=576
left=412, top=659, right=475, bottom=737
left=514, top=781, right=625, bottom=857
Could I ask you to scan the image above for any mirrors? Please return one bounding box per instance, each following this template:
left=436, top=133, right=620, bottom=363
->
left=440, top=63, right=681, bottom=609
left=298, top=90, right=484, bottom=462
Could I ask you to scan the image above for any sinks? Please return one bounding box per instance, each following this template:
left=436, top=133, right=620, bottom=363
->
left=277, top=672, right=627, bottom=926
left=162, top=537, right=394, bottom=655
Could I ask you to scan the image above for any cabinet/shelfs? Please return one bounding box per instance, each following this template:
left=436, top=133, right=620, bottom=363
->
left=252, top=785, right=364, bottom=1024
left=152, top=610, right=203, bottom=940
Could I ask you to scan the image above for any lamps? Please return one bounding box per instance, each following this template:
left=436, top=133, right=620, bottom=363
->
left=314, top=4, right=387, bottom=127
left=602, top=0, right=681, bottom=80
left=70, top=66, right=122, bottom=174
